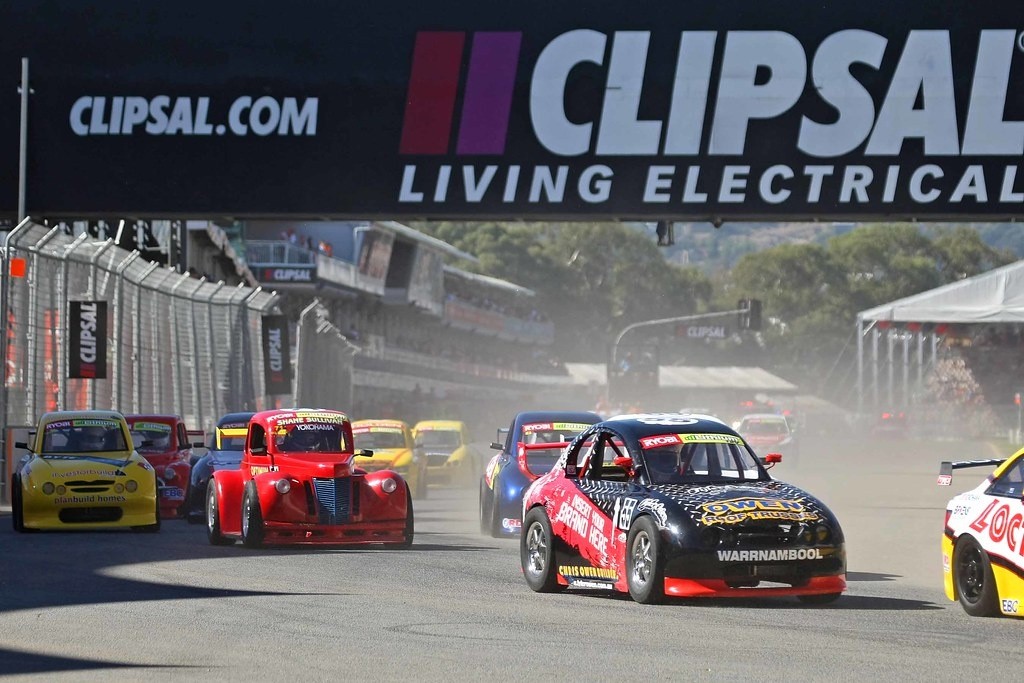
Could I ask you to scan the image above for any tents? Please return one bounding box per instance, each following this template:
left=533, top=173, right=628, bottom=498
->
left=820, top=261, right=1024, bottom=412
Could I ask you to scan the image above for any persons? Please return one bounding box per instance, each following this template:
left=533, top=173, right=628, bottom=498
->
left=77, top=427, right=105, bottom=450
left=287, top=425, right=320, bottom=449
left=144, top=431, right=169, bottom=448
left=646, top=441, right=679, bottom=480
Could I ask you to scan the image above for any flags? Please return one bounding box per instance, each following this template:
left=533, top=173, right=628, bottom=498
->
left=67, top=300, right=107, bottom=379
left=263, top=314, right=290, bottom=394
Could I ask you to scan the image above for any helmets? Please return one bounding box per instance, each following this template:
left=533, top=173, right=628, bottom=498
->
left=647, top=445, right=680, bottom=482
left=289, top=425, right=321, bottom=451
left=146, top=429, right=169, bottom=448
left=79, top=426, right=107, bottom=451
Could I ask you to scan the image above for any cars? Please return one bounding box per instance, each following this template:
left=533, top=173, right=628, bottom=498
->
left=727, top=411, right=796, bottom=462
left=937, top=445, right=1023, bottom=621
left=341, top=417, right=428, bottom=497
left=410, top=420, right=484, bottom=495
left=9, top=411, right=161, bottom=531
left=187, top=412, right=256, bottom=525
left=121, top=413, right=206, bottom=521
left=479, top=410, right=602, bottom=539
left=520, top=414, right=848, bottom=609
left=204, top=407, right=414, bottom=548
